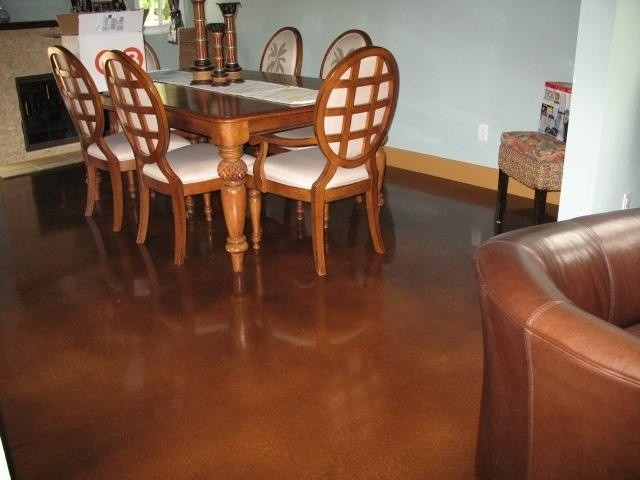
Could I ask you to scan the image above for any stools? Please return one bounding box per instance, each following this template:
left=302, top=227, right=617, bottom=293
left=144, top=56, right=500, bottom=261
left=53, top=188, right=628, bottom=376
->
left=493, top=131, right=567, bottom=233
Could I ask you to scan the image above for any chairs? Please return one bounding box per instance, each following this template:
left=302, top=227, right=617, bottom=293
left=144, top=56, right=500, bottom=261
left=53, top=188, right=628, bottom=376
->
left=259, top=26, right=303, bottom=77
left=320, top=30, right=373, bottom=79
left=244, top=47, right=401, bottom=281
left=99, top=49, right=248, bottom=265
left=47, top=44, right=192, bottom=232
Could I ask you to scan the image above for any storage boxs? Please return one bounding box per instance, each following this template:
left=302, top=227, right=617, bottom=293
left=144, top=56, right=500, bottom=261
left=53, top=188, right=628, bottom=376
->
left=539, top=82, right=571, bottom=145
left=56, top=8, right=148, bottom=93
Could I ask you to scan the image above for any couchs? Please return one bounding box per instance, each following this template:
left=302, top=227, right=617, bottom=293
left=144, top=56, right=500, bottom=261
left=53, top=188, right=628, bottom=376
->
left=473, top=208, right=638, bottom=480
